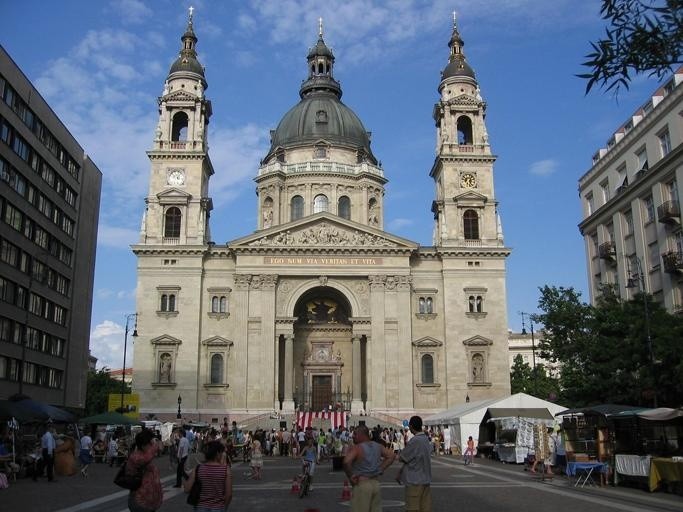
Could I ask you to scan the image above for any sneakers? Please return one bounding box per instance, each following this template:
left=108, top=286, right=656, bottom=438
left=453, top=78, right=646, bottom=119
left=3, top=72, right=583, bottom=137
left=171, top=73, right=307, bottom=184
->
left=30, top=474, right=58, bottom=483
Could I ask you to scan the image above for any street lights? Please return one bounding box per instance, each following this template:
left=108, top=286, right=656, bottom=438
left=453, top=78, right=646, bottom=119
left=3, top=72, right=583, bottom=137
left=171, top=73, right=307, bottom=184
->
left=118, top=312, right=141, bottom=416
left=623, top=252, right=660, bottom=408
left=176, top=394, right=183, bottom=419
left=519, top=310, right=538, bottom=397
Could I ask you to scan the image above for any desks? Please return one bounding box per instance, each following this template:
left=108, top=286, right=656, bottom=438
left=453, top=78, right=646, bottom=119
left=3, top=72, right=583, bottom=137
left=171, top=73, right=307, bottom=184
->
left=566, top=462, right=608, bottom=488
left=649, top=458, right=683, bottom=492
left=614, top=454, right=653, bottom=487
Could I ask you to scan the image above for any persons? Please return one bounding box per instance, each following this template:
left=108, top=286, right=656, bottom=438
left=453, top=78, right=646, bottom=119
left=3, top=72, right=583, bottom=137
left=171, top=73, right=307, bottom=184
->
left=0, top=437, right=20, bottom=483
left=79, top=429, right=95, bottom=480
left=464, top=436, right=475, bottom=464
left=250, top=435, right=264, bottom=479
left=342, top=416, right=432, bottom=512
left=127, top=429, right=232, bottom=512
left=247, top=219, right=400, bottom=249
left=296, top=435, right=317, bottom=492
left=32, top=423, right=77, bottom=482
left=529, top=427, right=566, bottom=476
left=73, top=417, right=452, bottom=468
left=296, top=399, right=346, bottom=411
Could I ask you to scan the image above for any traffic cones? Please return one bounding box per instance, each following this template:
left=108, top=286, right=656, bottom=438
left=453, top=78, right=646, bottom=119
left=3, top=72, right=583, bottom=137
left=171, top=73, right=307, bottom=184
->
left=341, top=478, right=352, bottom=501
left=290, top=475, right=300, bottom=495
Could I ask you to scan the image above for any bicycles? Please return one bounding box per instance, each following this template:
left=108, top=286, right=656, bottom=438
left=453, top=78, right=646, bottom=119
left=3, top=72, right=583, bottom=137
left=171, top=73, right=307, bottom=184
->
left=462, top=446, right=476, bottom=466
left=300, top=457, right=319, bottom=497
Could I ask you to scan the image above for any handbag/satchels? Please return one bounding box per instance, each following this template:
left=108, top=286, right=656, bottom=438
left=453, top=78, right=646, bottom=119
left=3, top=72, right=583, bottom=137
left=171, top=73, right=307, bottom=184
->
left=186, top=478, right=201, bottom=505
left=114, top=462, right=143, bottom=490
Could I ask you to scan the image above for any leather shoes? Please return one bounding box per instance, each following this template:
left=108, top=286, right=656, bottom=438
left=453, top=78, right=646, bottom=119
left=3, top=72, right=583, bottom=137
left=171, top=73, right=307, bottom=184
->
left=172, top=484, right=181, bottom=488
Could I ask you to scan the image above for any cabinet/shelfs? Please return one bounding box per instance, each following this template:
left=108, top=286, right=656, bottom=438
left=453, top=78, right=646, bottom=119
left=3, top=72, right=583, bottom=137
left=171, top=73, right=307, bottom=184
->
left=117, top=434, right=135, bottom=465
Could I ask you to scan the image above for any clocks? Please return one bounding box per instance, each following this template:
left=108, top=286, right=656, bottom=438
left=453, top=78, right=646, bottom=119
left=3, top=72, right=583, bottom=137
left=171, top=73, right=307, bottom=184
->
left=458, top=170, right=478, bottom=190
left=166, top=167, right=186, bottom=188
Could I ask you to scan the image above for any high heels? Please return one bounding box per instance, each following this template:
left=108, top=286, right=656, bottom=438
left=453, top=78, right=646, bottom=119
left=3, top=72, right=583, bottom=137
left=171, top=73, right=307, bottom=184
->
left=81, top=469, right=88, bottom=480
